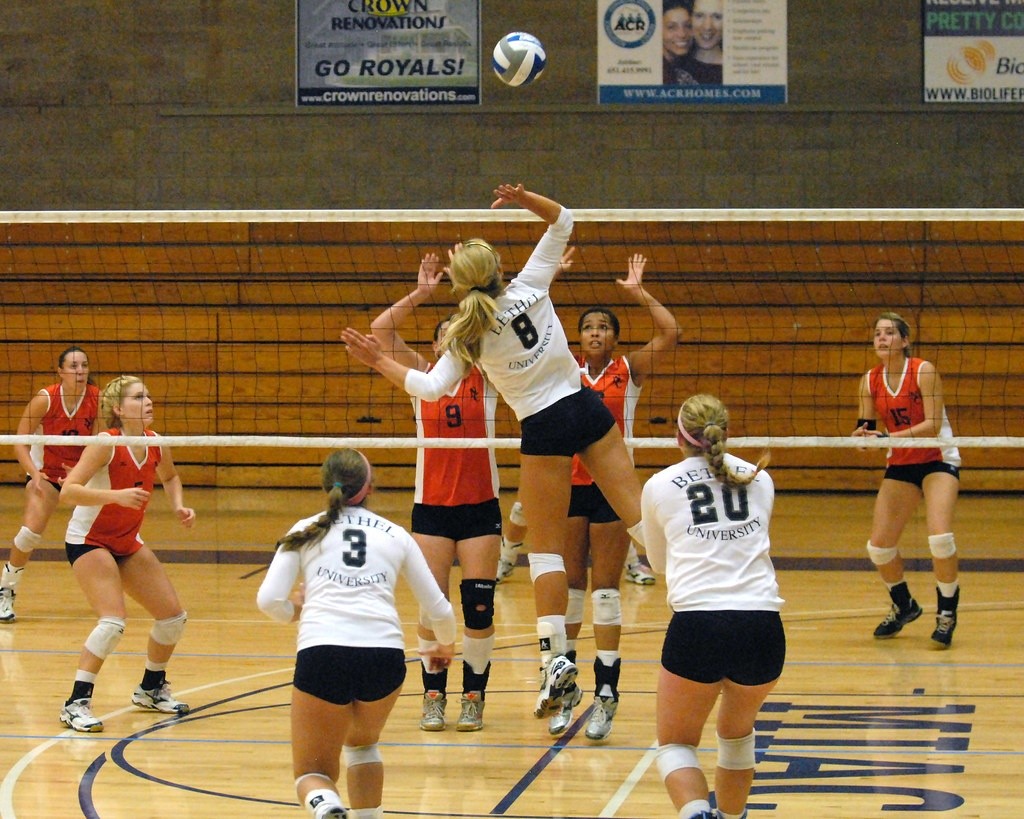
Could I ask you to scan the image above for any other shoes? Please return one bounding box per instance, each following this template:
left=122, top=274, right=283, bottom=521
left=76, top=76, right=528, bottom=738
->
left=314, top=804, right=348, bottom=819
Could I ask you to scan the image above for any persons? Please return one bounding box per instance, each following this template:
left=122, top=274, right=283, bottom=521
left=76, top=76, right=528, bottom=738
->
left=850, top=312, right=961, bottom=650
left=340, top=184, right=684, bottom=739
left=0, top=348, right=108, bottom=623
left=256, top=449, right=456, bottom=819
left=58, top=374, right=191, bottom=734
left=639, top=394, right=786, bottom=819
left=663, top=0, right=722, bottom=85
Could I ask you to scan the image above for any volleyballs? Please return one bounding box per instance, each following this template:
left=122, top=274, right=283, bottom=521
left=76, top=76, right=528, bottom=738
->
left=492, top=31, right=547, bottom=88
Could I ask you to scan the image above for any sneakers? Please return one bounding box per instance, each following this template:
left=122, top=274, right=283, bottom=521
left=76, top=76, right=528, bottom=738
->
left=626, top=565, right=656, bottom=585
left=547, top=685, right=583, bottom=734
left=420, top=691, right=447, bottom=731
left=872, top=601, right=922, bottom=638
left=0, top=588, right=15, bottom=623
left=585, top=694, right=618, bottom=740
left=932, top=605, right=957, bottom=645
left=533, top=655, right=578, bottom=720
left=59, top=697, right=104, bottom=733
left=456, top=692, right=485, bottom=731
left=496, top=558, right=517, bottom=584
left=133, top=683, right=189, bottom=714
